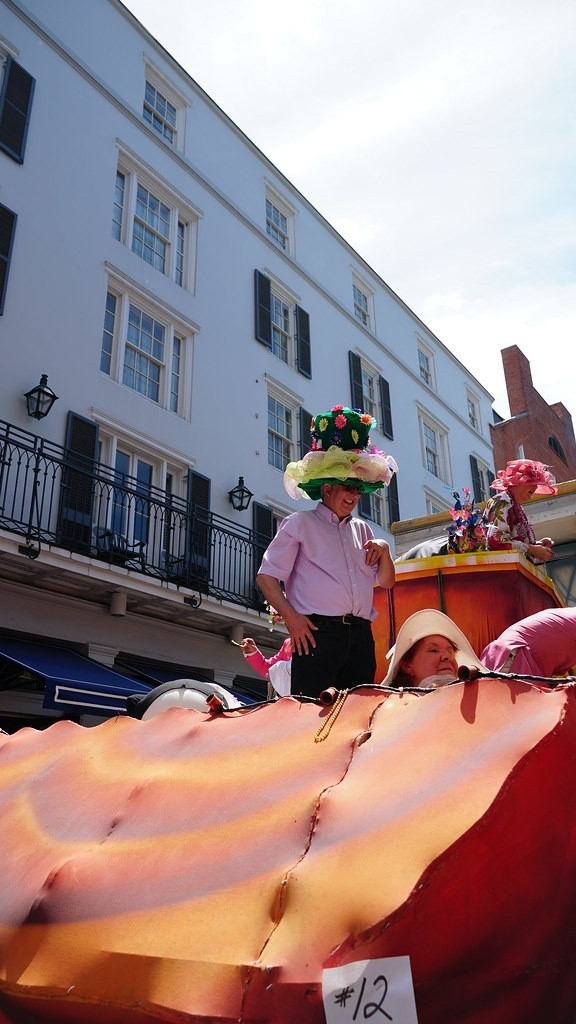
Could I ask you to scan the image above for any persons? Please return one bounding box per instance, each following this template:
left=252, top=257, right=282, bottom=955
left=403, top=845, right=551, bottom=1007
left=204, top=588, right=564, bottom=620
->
left=239, top=638, right=292, bottom=697
left=479, top=608, right=576, bottom=677
left=479, top=460, right=558, bottom=561
left=381, top=609, right=491, bottom=688
left=256, top=404, right=395, bottom=698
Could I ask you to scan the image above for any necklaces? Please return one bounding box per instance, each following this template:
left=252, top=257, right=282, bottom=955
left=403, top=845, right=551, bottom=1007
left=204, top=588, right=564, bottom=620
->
left=508, top=488, right=536, bottom=544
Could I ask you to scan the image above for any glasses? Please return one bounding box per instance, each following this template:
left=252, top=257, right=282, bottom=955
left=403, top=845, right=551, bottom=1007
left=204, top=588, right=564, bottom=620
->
left=338, top=483, right=364, bottom=495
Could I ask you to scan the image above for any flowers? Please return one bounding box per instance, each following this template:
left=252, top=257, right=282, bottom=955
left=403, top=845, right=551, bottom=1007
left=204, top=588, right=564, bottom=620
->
left=264, top=600, right=286, bottom=632
left=309, top=405, right=385, bottom=458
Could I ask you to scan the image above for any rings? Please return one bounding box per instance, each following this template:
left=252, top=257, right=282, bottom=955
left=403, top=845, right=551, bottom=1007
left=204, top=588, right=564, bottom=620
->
left=374, top=550, right=378, bottom=555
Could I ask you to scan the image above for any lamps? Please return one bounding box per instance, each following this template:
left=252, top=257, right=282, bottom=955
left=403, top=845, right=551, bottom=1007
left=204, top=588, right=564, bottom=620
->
left=228, top=477, right=254, bottom=511
left=24, top=374, right=59, bottom=421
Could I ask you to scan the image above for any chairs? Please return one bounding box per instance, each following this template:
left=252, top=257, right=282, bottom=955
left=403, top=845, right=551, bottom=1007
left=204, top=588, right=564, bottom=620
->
left=94, top=526, right=145, bottom=572
left=162, top=551, right=214, bottom=594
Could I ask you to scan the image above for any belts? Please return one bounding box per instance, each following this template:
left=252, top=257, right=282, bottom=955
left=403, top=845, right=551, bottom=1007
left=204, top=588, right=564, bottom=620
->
left=306, top=613, right=372, bottom=625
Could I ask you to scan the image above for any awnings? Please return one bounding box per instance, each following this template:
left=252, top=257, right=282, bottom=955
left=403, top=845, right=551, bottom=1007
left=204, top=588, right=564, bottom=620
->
left=118, top=657, right=280, bottom=707
left=1, top=638, right=154, bottom=716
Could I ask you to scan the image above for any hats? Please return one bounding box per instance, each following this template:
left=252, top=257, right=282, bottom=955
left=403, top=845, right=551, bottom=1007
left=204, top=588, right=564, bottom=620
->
left=380, top=609, right=490, bottom=687
left=489, top=459, right=559, bottom=496
left=284, top=405, right=399, bottom=500
